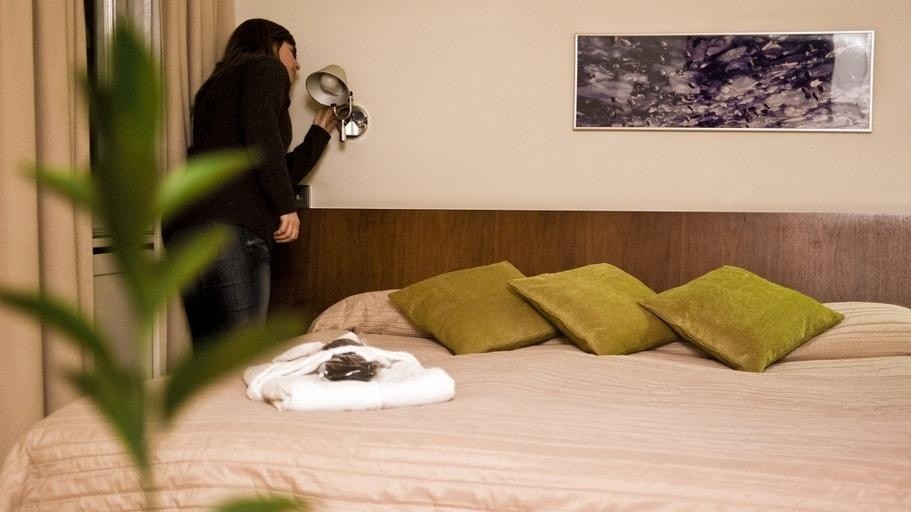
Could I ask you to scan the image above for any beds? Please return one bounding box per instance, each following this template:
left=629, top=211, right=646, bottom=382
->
left=1, top=208, right=911, bottom=512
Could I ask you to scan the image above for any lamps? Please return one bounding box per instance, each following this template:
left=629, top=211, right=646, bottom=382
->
left=305, top=65, right=368, bottom=143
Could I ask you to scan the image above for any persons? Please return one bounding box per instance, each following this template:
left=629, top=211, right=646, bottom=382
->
left=161, top=17, right=341, bottom=360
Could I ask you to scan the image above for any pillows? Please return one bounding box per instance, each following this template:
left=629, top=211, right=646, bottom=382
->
left=637, top=265, right=845, bottom=373
left=507, top=262, right=680, bottom=355
left=388, top=259, right=559, bottom=356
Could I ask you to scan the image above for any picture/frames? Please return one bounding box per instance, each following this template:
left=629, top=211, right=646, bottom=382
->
left=572, top=29, right=875, bottom=133
